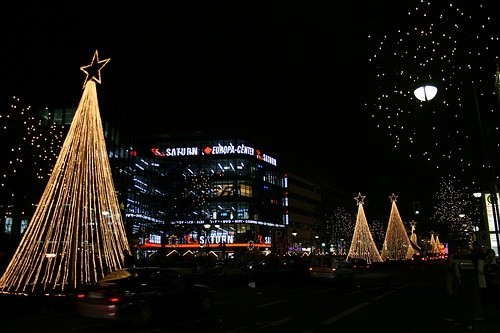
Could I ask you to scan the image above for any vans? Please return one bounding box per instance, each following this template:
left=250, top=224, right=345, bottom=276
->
left=452, top=252, right=474, bottom=271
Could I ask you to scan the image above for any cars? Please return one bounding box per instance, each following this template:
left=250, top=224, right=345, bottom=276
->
left=353, top=260, right=377, bottom=273
left=75, top=268, right=219, bottom=325
left=241, top=250, right=351, bottom=281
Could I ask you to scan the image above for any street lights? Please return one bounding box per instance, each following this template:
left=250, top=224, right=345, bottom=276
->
left=472, top=184, right=500, bottom=265
